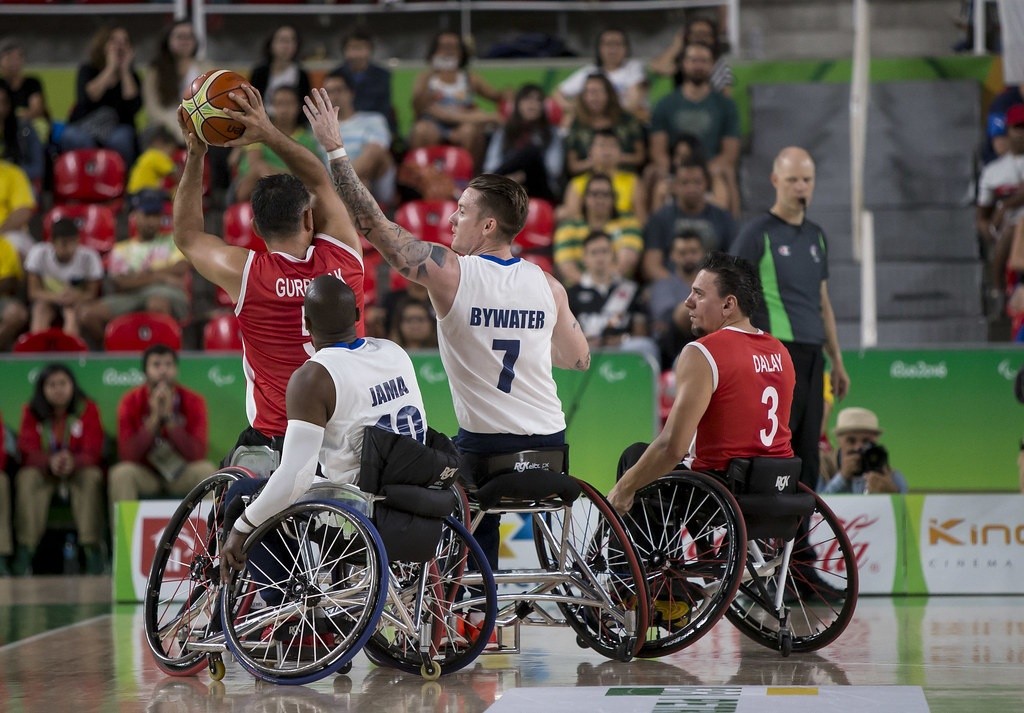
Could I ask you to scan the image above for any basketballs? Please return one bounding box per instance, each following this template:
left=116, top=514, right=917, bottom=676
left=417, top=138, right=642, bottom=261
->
left=182, top=69, right=252, bottom=146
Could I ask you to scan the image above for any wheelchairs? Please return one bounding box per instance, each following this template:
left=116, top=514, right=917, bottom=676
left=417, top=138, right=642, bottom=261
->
left=582, top=453, right=858, bottom=655
left=332, top=446, right=652, bottom=679
left=147, top=444, right=497, bottom=686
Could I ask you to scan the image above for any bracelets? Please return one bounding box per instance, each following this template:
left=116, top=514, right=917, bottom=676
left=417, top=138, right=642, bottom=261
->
left=328, top=148, right=346, bottom=161
left=234, top=517, right=253, bottom=534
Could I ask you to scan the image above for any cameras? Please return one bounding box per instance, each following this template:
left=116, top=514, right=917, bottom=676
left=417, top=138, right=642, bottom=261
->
left=853, top=444, right=888, bottom=476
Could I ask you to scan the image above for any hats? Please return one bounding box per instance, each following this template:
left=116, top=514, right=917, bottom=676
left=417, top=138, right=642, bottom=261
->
left=1005, top=105, right=1024, bottom=127
left=50, top=216, right=78, bottom=240
left=127, top=191, right=159, bottom=214
left=987, top=113, right=1006, bottom=140
left=832, top=406, right=884, bottom=434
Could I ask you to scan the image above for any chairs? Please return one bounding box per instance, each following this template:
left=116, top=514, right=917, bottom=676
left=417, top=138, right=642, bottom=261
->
left=12, top=148, right=559, bottom=354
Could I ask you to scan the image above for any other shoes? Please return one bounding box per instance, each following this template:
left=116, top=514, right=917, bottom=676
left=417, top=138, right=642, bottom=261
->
left=227, top=622, right=337, bottom=661
left=10, top=543, right=32, bottom=577
left=398, top=621, right=468, bottom=651
left=464, top=612, right=498, bottom=651
left=597, top=589, right=689, bottom=632
left=786, top=564, right=849, bottom=605
left=81, top=542, right=104, bottom=575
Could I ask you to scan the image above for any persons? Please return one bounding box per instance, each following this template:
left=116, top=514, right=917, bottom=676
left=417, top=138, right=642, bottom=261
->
left=974, top=82, right=1024, bottom=341
left=815, top=406, right=906, bottom=494
left=105, top=342, right=218, bottom=536
left=727, top=144, right=846, bottom=607
left=300, top=88, right=590, bottom=649
left=605, top=250, right=796, bottom=633
left=14, top=363, right=109, bottom=575
left=171, top=83, right=364, bottom=524
left=0, top=13, right=751, bottom=368
left=219, top=274, right=427, bottom=648
